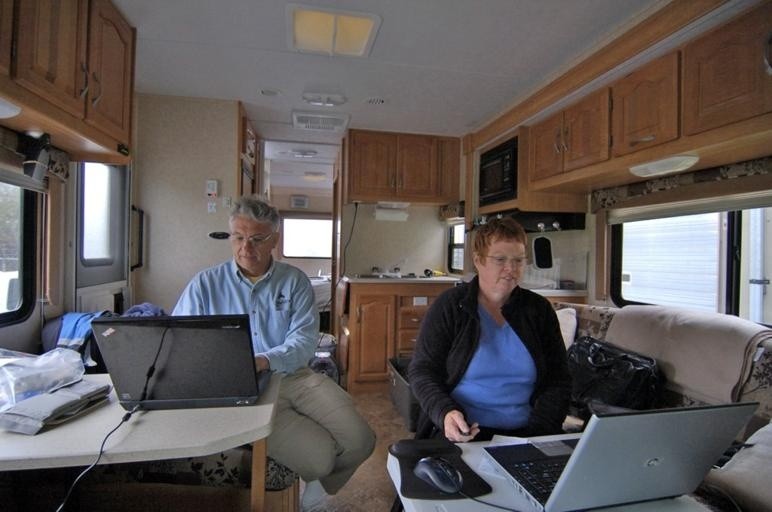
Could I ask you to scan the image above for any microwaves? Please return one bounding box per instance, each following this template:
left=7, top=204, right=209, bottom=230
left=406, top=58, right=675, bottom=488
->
left=478, top=134, right=519, bottom=207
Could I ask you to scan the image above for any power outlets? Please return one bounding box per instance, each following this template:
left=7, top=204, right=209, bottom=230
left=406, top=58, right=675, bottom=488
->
left=353, top=200, right=360, bottom=204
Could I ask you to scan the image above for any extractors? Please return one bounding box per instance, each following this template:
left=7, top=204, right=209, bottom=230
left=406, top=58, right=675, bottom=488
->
left=475, top=208, right=586, bottom=231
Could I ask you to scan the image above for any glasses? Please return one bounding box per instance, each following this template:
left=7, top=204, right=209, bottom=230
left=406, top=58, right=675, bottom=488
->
left=228, top=233, right=273, bottom=244
left=478, top=253, right=528, bottom=267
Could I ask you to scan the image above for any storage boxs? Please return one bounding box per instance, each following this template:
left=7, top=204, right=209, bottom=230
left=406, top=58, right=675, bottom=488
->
left=384, top=357, right=419, bottom=432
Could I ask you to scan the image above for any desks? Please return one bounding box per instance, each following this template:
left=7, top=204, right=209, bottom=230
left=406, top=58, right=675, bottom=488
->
left=0, top=368, right=279, bottom=512
left=389, top=442, right=717, bottom=510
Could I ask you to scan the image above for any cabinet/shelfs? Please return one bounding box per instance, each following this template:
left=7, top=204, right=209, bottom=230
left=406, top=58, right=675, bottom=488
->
left=351, top=284, right=456, bottom=394
left=239, top=101, right=259, bottom=165
left=620, top=1, right=771, bottom=177
left=345, top=130, right=461, bottom=205
left=0, top=0, right=137, bottom=165
left=529, top=93, right=640, bottom=190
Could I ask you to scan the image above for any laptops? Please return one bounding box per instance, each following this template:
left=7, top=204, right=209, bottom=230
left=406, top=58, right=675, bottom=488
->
left=91, top=313, right=272, bottom=411
left=480, top=401, right=760, bottom=512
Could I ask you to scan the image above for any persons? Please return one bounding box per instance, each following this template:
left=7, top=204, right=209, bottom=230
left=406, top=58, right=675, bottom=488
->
left=403, top=217, right=573, bottom=442
left=170, top=192, right=377, bottom=511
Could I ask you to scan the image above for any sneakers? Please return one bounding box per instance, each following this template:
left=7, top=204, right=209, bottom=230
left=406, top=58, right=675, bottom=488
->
left=299, top=479, right=330, bottom=510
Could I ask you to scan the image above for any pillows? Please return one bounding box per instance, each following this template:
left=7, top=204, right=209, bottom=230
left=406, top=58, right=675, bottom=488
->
left=555, top=306, right=579, bottom=349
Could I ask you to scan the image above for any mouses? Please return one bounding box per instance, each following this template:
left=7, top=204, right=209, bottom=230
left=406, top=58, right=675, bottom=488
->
left=412, top=455, right=463, bottom=494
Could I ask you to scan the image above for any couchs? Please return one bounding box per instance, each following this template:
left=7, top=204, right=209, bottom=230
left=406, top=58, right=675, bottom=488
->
left=552, top=297, right=772, bottom=512
left=11, top=313, right=342, bottom=512
